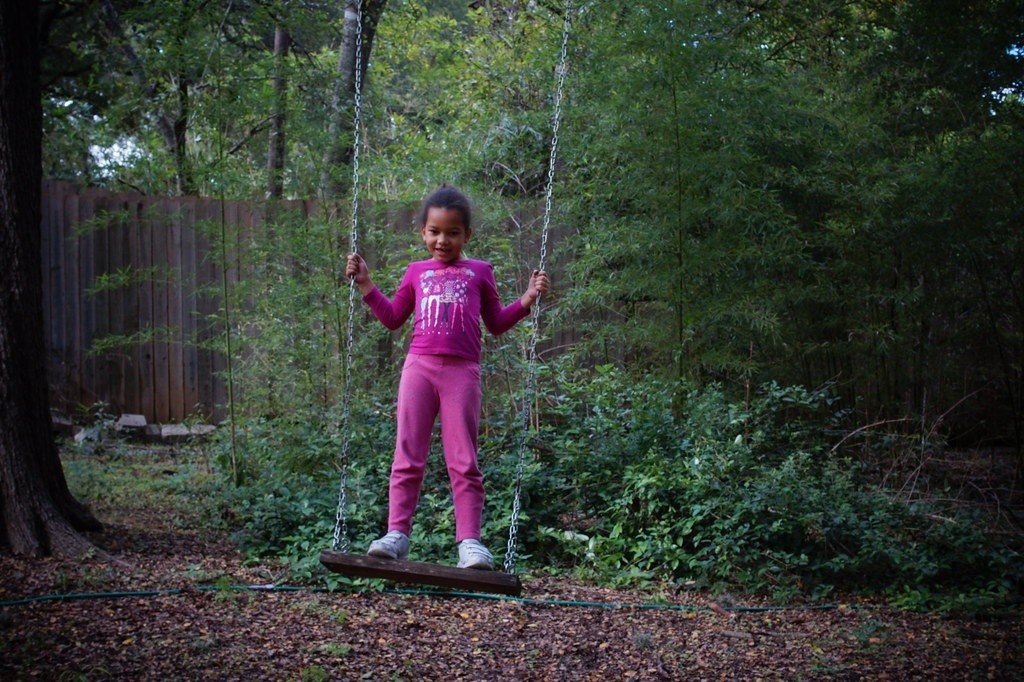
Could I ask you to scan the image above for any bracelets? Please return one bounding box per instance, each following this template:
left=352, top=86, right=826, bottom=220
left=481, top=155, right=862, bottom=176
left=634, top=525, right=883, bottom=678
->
left=362, top=284, right=374, bottom=294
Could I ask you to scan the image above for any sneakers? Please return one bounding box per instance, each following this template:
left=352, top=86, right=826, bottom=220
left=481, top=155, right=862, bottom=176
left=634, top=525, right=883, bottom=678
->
left=457, top=538, right=495, bottom=570
left=367, top=530, right=410, bottom=559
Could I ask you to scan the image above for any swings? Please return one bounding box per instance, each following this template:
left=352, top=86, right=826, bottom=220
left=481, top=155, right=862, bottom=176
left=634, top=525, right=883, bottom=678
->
left=321, top=0, right=570, bottom=596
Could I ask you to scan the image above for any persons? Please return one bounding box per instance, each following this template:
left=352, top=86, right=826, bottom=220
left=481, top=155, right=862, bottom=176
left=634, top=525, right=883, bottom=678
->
left=346, top=185, right=549, bottom=568
